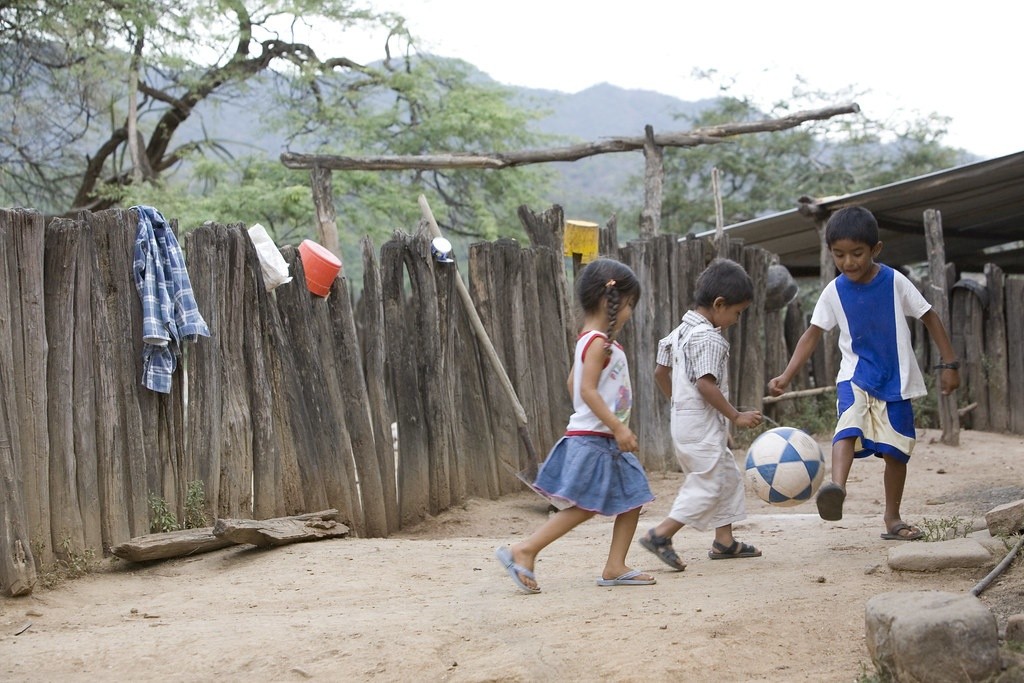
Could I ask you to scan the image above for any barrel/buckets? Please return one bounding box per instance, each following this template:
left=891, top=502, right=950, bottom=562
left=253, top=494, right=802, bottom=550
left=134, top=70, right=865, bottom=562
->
left=561, top=220, right=599, bottom=265
left=297, top=239, right=340, bottom=297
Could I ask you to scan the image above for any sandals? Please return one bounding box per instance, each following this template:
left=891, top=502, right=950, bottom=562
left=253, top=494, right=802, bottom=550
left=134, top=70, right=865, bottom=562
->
left=640, top=529, right=688, bottom=570
left=708, top=539, right=763, bottom=558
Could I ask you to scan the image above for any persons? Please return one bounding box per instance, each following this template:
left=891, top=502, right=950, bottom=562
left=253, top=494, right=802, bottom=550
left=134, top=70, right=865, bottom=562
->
left=768, top=207, right=960, bottom=541
left=497, top=259, right=657, bottom=594
left=639, top=260, right=764, bottom=572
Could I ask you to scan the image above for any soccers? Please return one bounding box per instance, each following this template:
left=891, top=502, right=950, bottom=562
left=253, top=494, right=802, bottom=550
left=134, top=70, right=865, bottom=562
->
left=744, top=426, right=825, bottom=507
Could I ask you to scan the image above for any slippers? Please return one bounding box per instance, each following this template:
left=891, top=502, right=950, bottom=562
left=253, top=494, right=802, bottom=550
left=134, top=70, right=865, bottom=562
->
left=880, top=524, right=925, bottom=540
left=497, top=546, right=541, bottom=593
left=817, top=483, right=844, bottom=521
left=596, top=570, right=657, bottom=586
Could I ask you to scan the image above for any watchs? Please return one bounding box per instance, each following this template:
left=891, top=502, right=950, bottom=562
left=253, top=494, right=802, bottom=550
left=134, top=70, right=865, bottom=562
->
left=932, top=362, right=961, bottom=372
